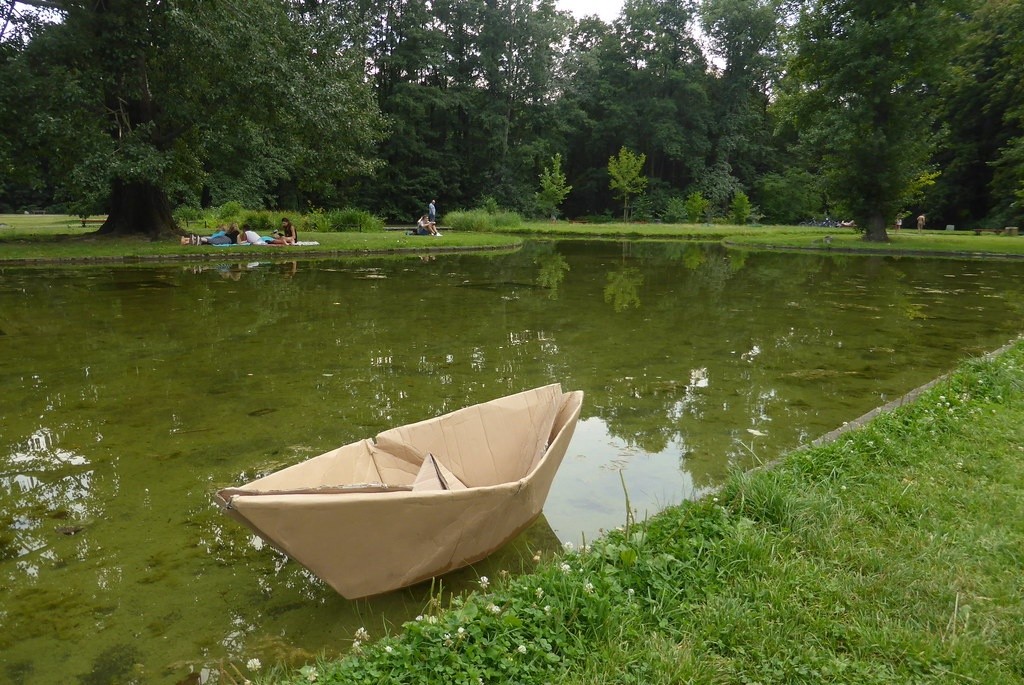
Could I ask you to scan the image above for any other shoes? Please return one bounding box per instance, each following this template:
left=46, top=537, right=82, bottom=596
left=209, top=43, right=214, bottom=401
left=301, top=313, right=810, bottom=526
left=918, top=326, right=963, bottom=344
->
left=192, top=234, right=196, bottom=246
left=435, top=231, right=442, bottom=236
left=196, top=235, right=201, bottom=246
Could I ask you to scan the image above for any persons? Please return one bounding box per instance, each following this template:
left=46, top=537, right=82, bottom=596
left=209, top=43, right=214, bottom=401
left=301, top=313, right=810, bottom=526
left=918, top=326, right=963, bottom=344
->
left=916, top=214, right=926, bottom=236
left=180, top=217, right=298, bottom=246
left=415, top=200, right=443, bottom=236
left=895, top=217, right=902, bottom=234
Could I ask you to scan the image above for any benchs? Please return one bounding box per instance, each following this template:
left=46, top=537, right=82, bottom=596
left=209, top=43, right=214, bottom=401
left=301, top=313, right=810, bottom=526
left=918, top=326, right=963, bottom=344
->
left=79, top=219, right=106, bottom=227
left=972, top=229, right=1004, bottom=236
